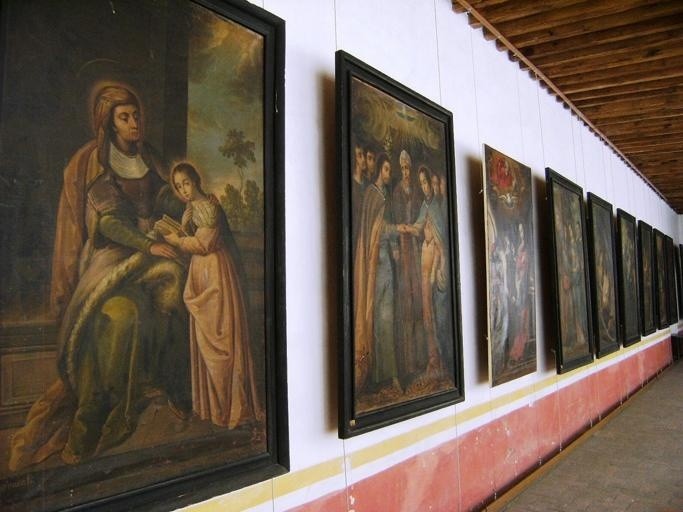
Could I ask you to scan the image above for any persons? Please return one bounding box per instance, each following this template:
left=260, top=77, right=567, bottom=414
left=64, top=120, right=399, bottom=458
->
left=46, top=79, right=193, bottom=465
left=488, top=218, right=532, bottom=377
left=162, top=158, right=263, bottom=431
left=351, top=142, right=456, bottom=410
left=555, top=212, right=589, bottom=346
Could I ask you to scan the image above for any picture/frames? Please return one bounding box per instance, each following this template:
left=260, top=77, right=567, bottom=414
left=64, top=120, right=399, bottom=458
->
left=586, top=192, right=620, bottom=359
left=617, top=208, right=641, bottom=348
left=485, top=144, right=537, bottom=386
left=546, top=167, right=594, bottom=375
left=0, top=0, right=290, bottom=512
left=335, top=49, right=465, bottom=440
left=638, top=219, right=683, bottom=337
